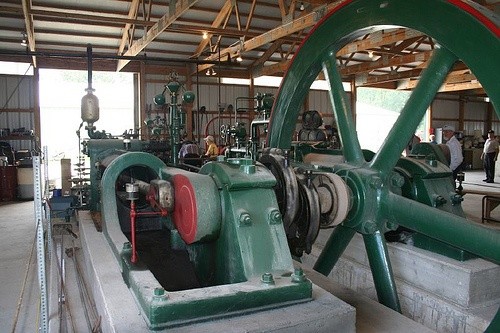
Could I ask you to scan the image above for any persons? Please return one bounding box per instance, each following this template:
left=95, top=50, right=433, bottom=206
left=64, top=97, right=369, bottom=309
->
left=203, top=134, right=219, bottom=158
left=429, top=134, right=437, bottom=143
left=439, top=125, right=464, bottom=191
left=480, top=129, right=499, bottom=182
left=179, top=141, right=200, bottom=170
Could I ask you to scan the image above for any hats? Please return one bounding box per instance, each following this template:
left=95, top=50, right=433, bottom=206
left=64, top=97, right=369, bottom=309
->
left=487, top=130, right=494, bottom=133
left=204, top=135, right=215, bottom=142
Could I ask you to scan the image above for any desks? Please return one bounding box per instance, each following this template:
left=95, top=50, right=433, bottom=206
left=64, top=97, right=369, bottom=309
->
left=462, top=147, right=483, bottom=169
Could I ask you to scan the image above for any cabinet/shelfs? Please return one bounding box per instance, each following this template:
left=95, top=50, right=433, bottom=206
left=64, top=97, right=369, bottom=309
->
left=0, top=165, right=17, bottom=202
left=0, top=136, right=34, bottom=167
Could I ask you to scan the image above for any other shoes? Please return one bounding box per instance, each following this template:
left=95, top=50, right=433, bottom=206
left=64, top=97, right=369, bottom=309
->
left=483, top=177, right=489, bottom=181
left=487, top=179, right=494, bottom=183
